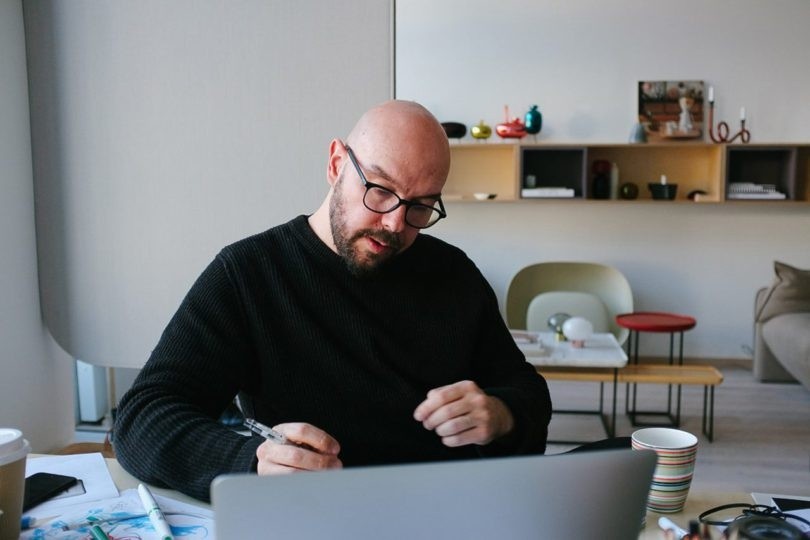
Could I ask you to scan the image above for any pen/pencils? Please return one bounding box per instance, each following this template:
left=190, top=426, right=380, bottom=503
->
left=243, top=418, right=296, bottom=446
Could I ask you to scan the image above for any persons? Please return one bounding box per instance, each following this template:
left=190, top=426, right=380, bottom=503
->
left=111, top=99, right=553, bottom=504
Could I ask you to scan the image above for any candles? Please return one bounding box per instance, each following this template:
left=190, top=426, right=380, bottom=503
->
left=741, top=107, right=746, bottom=119
left=708, top=87, right=714, bottom=101
left=661, top=173, right=666, bottom=186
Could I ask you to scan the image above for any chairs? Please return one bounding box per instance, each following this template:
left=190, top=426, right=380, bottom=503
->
left=503, top=261, right=634, bottom=450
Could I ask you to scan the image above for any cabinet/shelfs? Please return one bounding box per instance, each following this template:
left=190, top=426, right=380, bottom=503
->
left=441, top=143, right=810, bottom=205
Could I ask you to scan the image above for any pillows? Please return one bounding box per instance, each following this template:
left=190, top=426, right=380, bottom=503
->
left=524, top=291, right=610, bottom=335
left=755, top=260, right=810, bottom=322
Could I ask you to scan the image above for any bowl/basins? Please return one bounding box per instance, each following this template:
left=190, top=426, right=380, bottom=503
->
left=648, top=183, right=678, bottom=201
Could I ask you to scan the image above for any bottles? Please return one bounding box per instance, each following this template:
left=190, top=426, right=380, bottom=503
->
left=104, top=407, right=117, bottom=458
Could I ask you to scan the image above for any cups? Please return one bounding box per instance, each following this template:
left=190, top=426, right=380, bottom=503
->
left=632, top=427, right=698, bottom=513
left=592, top=161, right=620, bottom=201
left=0, top=428, right=29, bottom=540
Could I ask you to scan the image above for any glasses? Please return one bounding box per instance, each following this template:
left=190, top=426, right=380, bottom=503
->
left=344, top=145, right=447, bottom=229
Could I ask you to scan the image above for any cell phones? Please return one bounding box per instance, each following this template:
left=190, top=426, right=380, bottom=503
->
left=22, top=473, right=77, bottom=513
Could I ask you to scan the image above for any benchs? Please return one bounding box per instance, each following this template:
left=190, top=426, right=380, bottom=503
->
left=753, top=286, right=810, bottom=391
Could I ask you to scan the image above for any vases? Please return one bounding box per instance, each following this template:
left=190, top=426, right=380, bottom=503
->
left=648, top=184, right=677, bottom=200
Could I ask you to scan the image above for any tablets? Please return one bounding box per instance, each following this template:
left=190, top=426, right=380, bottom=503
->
left=751, top=493, right=810, bottom=513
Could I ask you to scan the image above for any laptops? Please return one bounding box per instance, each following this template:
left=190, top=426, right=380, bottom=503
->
left=210, top=449, right=658, bottom=540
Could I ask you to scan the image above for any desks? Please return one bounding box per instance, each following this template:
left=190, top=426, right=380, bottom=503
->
left=615, top=311, right=697, bottom=429
left=26, top=451, right=761, bottom=540
left=510, top=329, right=725, bottom=445
left=645, top=123, right=700, bottom=138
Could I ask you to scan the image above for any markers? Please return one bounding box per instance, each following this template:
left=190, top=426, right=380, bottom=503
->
left=90, top=526, right=108, bottom=540
left=138, top=483, right=174, bottom=540
